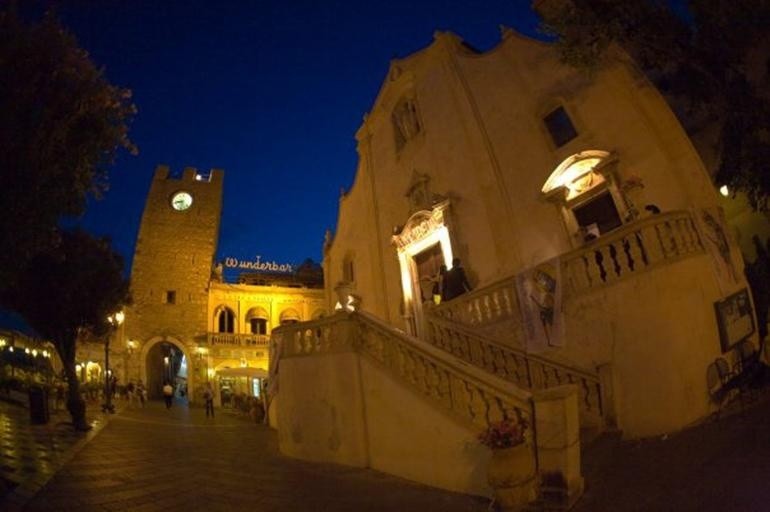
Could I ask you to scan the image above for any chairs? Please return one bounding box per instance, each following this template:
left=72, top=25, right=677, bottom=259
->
left=716, top=340, right=754, bottom=420
left=738, top=341, right=770, bottom=391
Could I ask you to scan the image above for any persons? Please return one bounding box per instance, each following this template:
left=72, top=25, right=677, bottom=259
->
left=644, top=203, right=678, bottom=256
left=435, top=265, right=449, bottom=302
left=441, top=256, right=474, bottom=302
left=52, top=375, right=218, bottom=419
left=577, top=226, right=608, bottom=280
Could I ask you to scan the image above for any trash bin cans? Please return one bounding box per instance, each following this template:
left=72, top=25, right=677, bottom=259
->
left=29, top=385, right=49, bottom=425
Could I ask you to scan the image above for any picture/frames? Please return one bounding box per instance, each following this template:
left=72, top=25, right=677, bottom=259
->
left=713, top=286, right=755, bottom=353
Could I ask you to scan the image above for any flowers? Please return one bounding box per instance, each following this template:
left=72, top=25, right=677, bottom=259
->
left=477, top=418, right=529, bottom=450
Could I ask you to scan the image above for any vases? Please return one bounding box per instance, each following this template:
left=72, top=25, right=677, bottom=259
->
left=486, top=441, right=536, bottom=490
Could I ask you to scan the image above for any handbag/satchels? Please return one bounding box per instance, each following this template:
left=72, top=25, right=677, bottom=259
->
left=203, top=392, right=210, bottom=399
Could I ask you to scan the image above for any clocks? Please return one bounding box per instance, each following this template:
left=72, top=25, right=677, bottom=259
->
left=168, top=189, right=194, bottom=212
